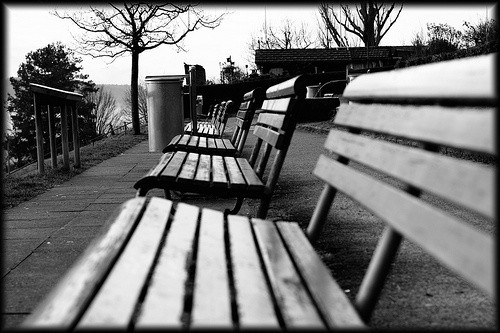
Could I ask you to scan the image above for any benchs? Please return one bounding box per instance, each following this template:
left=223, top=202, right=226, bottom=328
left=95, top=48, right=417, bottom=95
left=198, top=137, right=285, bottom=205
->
left=130, top=75, right=310, bottom=219
left=163, top=87, right=257, bottom=156
left=19, top=46, right=498, bottom=332
left=183, top=99, right=232, bottom=138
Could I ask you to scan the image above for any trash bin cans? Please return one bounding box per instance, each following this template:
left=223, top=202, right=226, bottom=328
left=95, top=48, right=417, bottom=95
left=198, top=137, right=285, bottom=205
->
left=144, top=72, right=188, bottom=153
